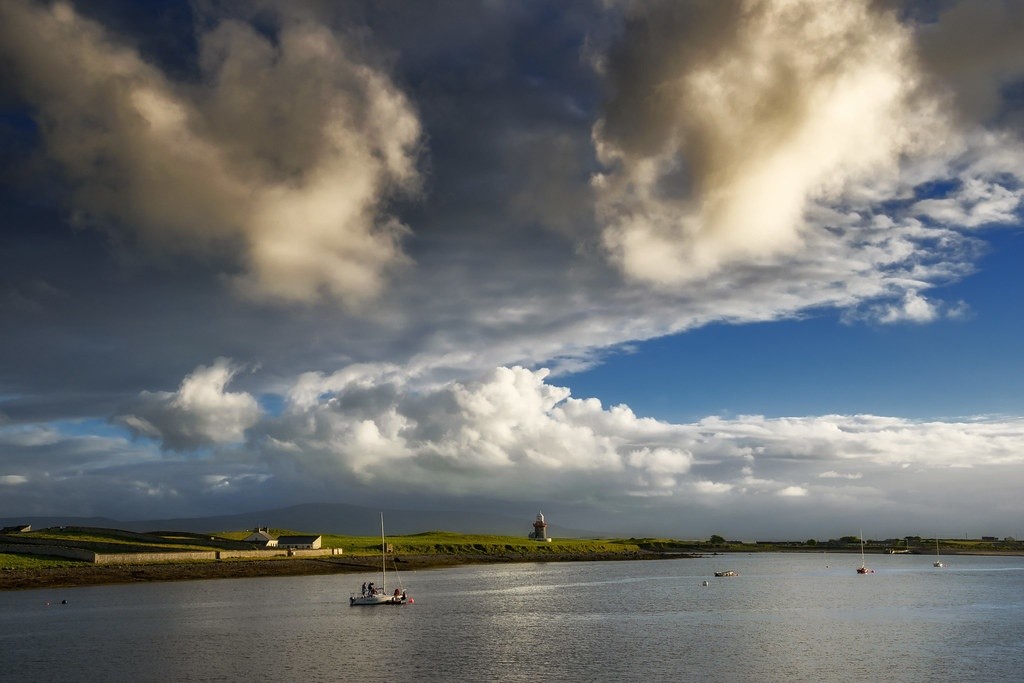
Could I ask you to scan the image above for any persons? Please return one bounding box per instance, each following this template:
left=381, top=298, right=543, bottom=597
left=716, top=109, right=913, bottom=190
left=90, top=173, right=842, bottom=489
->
left=362, top=582, right=374, bottom=596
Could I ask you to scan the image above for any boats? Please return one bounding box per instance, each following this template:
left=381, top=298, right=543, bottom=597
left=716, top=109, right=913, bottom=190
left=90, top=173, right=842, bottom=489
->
left=714, top=570, right=739, bottom=577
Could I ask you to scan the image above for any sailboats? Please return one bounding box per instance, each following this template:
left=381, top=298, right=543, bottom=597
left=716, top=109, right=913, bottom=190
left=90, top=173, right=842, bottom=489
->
left=348, top=512, right=407, bottom=605
left=856, top=530, right=870, bottom=574
left=932, top=534, right=943, bottom=568
